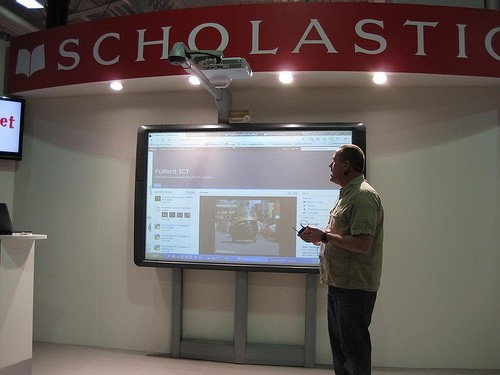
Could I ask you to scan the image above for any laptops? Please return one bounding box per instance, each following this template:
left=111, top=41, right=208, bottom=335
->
left=0, top=203, right=32, bottom=236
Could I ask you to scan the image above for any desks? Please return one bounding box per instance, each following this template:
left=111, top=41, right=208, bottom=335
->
left=0, top=233, right=48, bottom=375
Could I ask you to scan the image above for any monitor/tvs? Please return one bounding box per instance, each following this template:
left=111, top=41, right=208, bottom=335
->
left=0, top=95, right=26, bottom=161
left=133, top=121, right=367, bottom=274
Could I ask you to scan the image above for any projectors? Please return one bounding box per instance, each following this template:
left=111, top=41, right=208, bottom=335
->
left=183, top=57, right=252, bottom=82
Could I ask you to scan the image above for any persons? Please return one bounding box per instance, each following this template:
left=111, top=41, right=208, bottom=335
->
left=298, top=144, right=384, bottom=375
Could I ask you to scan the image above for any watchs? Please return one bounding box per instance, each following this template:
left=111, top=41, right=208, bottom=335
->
left=321, top=232, right=328, bottom=244
